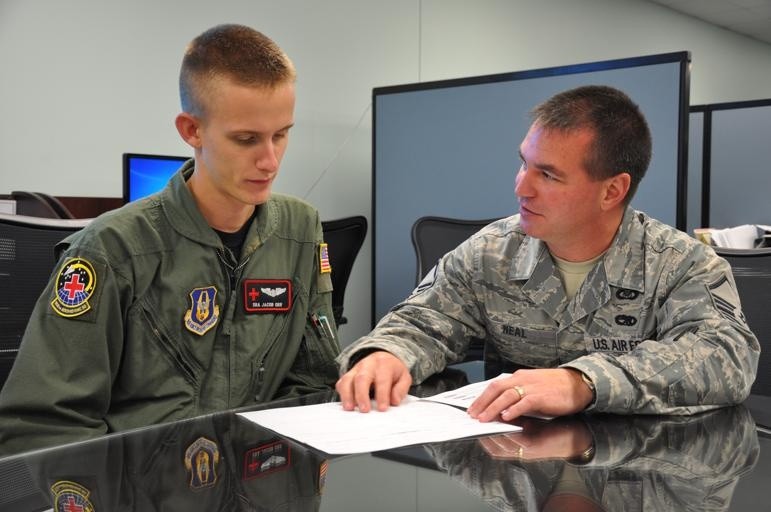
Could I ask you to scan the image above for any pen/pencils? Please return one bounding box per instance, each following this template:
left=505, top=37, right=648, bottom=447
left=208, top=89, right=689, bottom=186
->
left=308, top=311, right=334, bottom=338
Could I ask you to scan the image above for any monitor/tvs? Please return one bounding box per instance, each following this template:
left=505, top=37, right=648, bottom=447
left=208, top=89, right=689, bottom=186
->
left=121, top=153, right=190, bottom=205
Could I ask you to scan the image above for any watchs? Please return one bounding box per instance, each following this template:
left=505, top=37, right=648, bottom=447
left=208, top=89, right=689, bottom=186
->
left=579, top=369, right=597, bottom=409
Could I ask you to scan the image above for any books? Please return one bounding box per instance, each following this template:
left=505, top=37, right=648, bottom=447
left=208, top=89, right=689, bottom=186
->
left=693, top=223, right=760, bottom=249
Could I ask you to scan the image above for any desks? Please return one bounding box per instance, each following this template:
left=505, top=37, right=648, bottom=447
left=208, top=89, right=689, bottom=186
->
left=0, top=376, right=771, bottom=512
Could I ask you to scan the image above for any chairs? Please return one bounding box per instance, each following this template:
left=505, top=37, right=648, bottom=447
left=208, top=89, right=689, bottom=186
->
left=707, top=244, right=771, bottom=396
left=323, top=215, right=366, bottom=328
left=412, top=214, right=520, bottom=348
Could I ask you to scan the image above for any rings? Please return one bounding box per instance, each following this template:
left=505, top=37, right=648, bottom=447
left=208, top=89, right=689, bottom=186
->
left=512, top=384, right=526, bottom=400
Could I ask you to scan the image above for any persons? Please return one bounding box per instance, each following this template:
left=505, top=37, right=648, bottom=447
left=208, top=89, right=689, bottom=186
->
left=0, top=396, right=337, bottom=512
left=421, top=404, right=762, bottom=510
left=334, top=84, right=762, bottom=421
left=0, top=22, right=347, bottom=459
